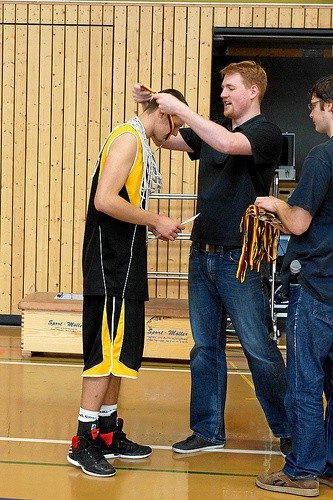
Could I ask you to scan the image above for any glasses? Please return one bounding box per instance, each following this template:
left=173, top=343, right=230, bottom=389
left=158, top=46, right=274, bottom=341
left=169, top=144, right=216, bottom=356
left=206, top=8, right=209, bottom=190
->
left=308, top=100, right=322, bottom=111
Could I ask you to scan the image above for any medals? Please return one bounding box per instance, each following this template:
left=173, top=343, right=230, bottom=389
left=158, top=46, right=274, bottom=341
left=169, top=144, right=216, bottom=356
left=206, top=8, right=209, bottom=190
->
left=156, top=141, right=165, bottom=151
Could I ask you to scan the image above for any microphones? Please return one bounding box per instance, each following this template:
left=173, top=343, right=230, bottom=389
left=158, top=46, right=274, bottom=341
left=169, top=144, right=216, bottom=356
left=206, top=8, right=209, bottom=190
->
left=290, top=260, right=301, bottom=285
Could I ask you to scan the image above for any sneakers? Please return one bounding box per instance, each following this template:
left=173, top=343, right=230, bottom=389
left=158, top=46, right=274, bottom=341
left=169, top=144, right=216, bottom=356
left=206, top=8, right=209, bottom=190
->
left=67, top=428, right=116, bottom=476
left=280, top=438, right=292, bottom=458
left=318, top=474, right=333, bottom=488
left=100, top=418, right=152, bottom=459
left=172, top=435, right=224, bottom=454
left=256, top=470, right=319, bottom=496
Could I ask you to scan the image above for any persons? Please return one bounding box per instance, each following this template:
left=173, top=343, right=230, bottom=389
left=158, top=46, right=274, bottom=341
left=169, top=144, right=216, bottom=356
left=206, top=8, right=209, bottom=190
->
left=66, top=88, right=190, bottom=478
left=253, top=74, right=333, bottom=497
left=131, top=59, right=293, bottom=458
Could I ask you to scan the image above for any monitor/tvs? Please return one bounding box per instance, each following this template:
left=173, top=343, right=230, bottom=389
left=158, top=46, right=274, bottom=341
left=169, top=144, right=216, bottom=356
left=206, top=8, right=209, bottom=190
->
left=276, top=132, right=295, bottom=169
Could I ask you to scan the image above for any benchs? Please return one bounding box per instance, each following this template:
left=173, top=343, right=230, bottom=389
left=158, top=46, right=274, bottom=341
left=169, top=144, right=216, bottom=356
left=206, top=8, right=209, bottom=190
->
left=18, top=292, right=195, bottom=360
